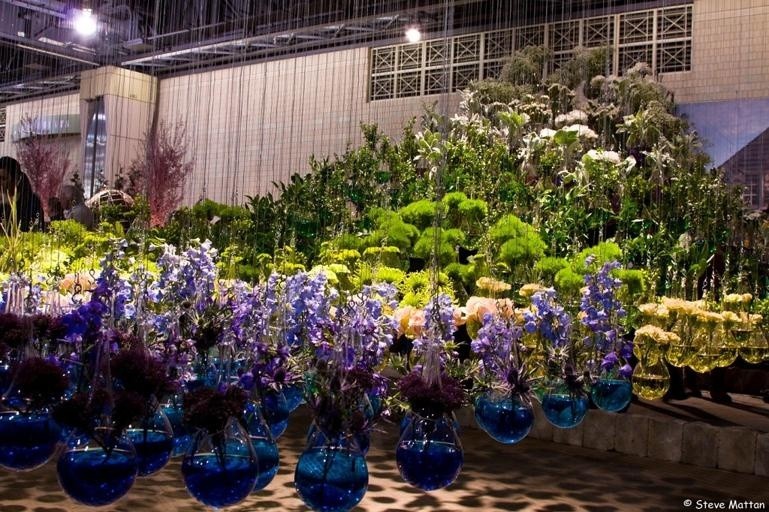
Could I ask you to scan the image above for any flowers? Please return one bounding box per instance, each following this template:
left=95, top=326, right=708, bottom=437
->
left=2, top=45, right=766, bottom=509
